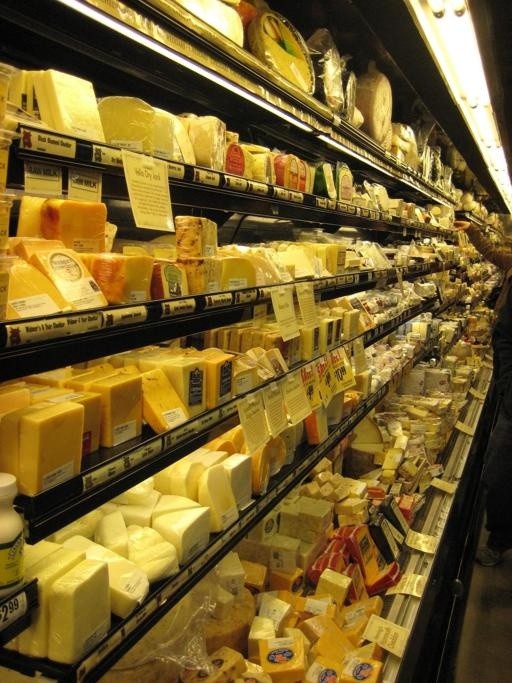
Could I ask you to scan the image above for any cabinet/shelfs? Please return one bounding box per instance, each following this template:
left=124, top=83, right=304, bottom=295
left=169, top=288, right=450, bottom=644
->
left=0, top=0, right=512, bottom=681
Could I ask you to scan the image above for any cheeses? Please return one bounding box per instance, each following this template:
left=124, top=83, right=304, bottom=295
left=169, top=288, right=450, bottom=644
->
left=0, top=0, right=492, bottom=683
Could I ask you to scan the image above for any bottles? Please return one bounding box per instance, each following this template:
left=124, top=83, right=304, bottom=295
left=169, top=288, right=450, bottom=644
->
left=1, top=473, right=25, bottom=600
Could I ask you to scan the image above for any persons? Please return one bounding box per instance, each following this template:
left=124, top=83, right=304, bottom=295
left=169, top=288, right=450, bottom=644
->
left=453, top=221, right=512, bottom=567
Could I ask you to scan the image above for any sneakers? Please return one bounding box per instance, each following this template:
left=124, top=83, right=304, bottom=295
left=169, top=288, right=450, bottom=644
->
left=477, top=545, right=508, bottom=566
